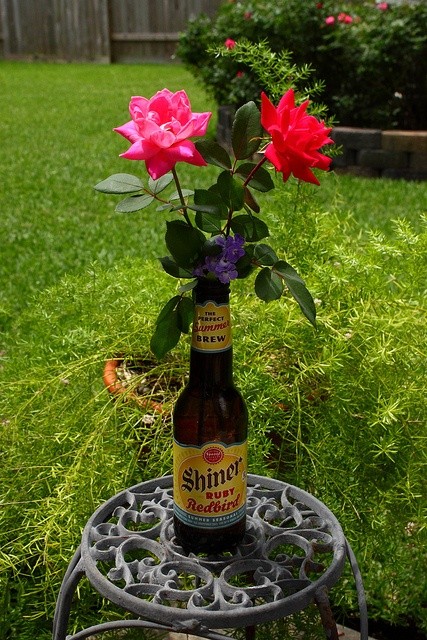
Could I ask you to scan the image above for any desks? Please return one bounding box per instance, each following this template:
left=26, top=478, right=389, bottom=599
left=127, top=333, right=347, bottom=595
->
left=52, top=469, right=368, bottom=640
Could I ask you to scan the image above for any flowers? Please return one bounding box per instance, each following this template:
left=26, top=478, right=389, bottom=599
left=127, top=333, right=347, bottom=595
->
left=88, top=86, right=335, bottom=359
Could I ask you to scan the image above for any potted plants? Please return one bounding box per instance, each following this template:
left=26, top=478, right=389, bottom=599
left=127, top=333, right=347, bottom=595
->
left=25, top=251, right=407, bottom=485
